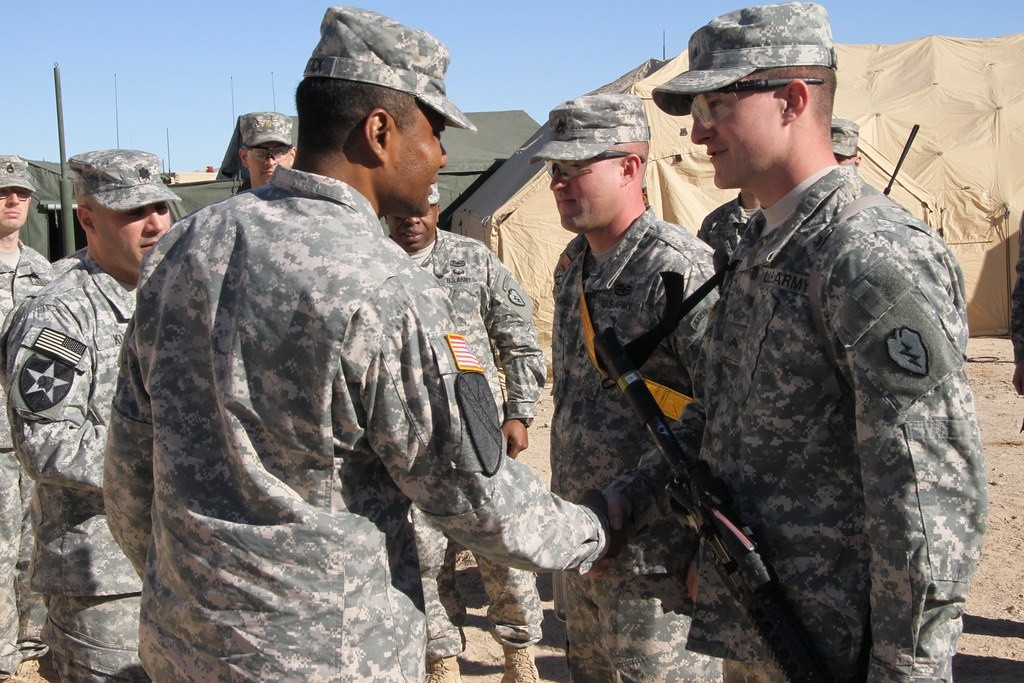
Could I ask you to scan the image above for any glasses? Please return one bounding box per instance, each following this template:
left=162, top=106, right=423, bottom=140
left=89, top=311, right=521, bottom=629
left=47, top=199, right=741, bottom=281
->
left=242, top=146, right=295, bottom=161
left=687, top=78, right=826, bottom=130
left=0, top=190, right=31, bottom=201
left=544, top=150, right=646, bottom=182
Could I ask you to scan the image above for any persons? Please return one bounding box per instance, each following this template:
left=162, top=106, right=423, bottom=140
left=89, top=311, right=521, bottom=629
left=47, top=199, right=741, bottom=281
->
left=101, top=9, right=622, bottom=683
left=0, top=94, right=861, bottom=683
left=602, top=3, right=988, bottom=683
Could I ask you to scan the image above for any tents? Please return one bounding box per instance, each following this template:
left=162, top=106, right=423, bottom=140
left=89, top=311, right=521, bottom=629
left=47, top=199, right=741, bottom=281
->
left=19, top=36, right=1024, bottom=387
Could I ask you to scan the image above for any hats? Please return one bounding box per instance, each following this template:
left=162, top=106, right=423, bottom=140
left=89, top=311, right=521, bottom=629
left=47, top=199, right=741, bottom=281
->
left=239, top=111, right=293, bottom=146
left=0, top=154, right=36, bottom=193
left=830, top=119, right=859, bottom=156
left=68, top=149, right=182, bottom=212
left=429, top=182, right=440, bottom=205
left=652, top=3, right=838, bottom=116
left=529, top=94, right=650, bottom=164
left=303, top=7, right=479, bottom=135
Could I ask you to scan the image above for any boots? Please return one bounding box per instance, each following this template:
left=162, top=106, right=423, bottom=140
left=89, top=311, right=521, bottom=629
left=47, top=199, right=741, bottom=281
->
left=501, top=644, right=540, bottom=683
left=425, top=656, right=461, bottom=683
